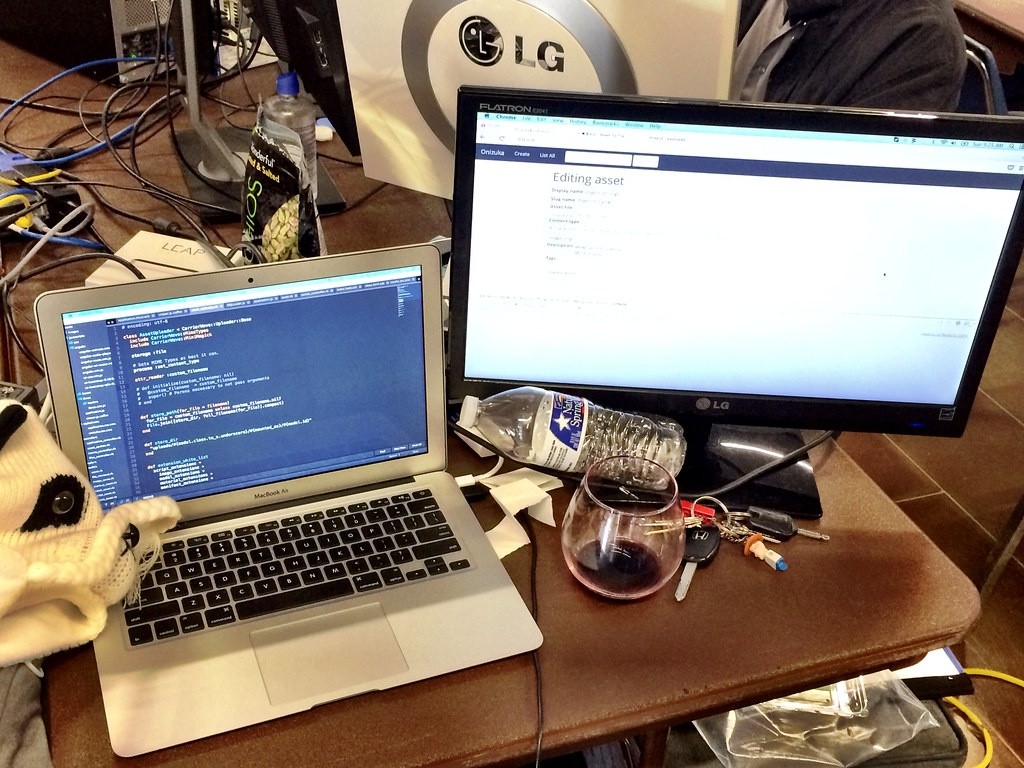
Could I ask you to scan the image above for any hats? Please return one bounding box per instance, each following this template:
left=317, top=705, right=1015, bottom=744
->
left=0, top=386, right=182, bottom=677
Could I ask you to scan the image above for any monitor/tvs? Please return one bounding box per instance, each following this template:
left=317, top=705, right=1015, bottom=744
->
left=169, top=0, right=1024, bottom=524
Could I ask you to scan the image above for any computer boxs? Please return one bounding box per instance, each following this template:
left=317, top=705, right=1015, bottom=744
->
left=214, top=0, right=279, bottom=75
left=0, top=0, right=214, bottom=90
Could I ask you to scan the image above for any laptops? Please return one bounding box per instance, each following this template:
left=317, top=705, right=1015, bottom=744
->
left=33, top=244, right=544, bottom=757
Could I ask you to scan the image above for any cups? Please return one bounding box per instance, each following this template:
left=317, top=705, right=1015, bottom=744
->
left=562, top=455, right=687, bottom=600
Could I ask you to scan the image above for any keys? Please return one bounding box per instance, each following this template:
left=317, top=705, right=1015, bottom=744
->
left=748, top=507, right=831, bottom=543
left=638, top=517, right=701, bottom=536
left=675, top=528, right=721, bottom=602
left=722, top=520, right=781, bottom=544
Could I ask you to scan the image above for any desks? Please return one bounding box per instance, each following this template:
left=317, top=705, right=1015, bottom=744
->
left=0, top=38, right=982, bottom=768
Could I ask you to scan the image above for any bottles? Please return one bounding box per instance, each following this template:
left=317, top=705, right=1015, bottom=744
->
left=459, top=385, right=688, bottom=490
left=265, top=72, right=316, bottom=197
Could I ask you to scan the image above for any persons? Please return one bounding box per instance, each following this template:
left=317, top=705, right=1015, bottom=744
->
left=732, top=0, right=967, bottom=110
